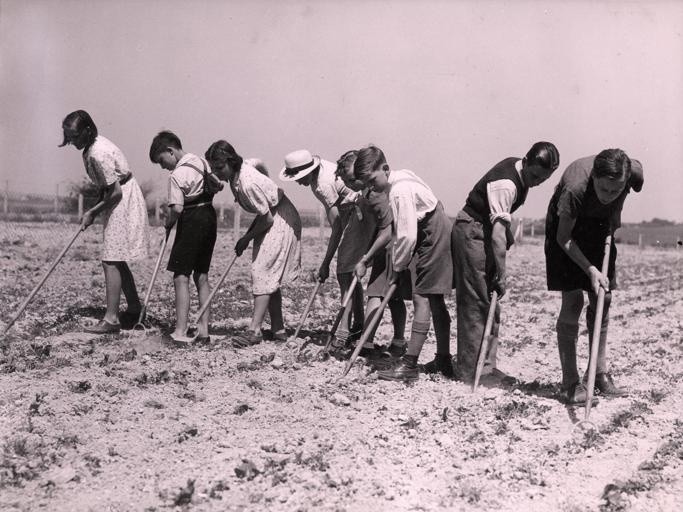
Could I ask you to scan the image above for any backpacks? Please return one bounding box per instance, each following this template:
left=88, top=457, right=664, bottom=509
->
left=204, top=170, right=224, bottom=195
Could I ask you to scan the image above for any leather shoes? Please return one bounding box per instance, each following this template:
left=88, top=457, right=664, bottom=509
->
left=419, top=353, right=453, bottom=376
left=376, top=362, right=419, bottom=382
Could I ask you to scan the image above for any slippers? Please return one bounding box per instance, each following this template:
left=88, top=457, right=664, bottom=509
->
left=204, top=170, right=224, bottom=195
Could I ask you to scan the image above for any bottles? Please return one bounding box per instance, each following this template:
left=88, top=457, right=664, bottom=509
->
left=279, top=150, right=321, bottom=182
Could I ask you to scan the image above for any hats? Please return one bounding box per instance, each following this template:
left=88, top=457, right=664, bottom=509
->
left=279, top=150, right=321, bottom=182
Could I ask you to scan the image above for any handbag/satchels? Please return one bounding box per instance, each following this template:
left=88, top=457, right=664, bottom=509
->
left=583, top=263, right=592, bottom=273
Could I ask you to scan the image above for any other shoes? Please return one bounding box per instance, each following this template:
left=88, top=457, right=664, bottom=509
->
left=191, top=337, right=211, bottom=348
left=89, top=320, right=120, bottom=333
left=342, top=345, right=376, bottom=361
left=262, top=329, right=287, bottom=341
left=559, top=382, right=599, bottom=406
left=120, top=312, right=146, bottom=328
left=466, top=368, right=516, bottom=389
left=150, top=335, right=188, bottom=348
left=231, top=330, right=263, bottom=347
left=582, top=373, right=626, bottom=396
left=381, top=338, right=407, bottom=360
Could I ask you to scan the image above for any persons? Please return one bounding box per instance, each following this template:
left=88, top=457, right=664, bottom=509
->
left=276, top=147, right=376, bottom=352
left=352, top=145, right=456, bottom=382
left=544, top=147, right=644, bottom=409
left=55, top=108, right=150, bottom=336
left=332, top=150, right=413, bottom=360
left=450, top=139, right=559, bottom=391
left=204, top=140, right=302, bottom=350
left=147, top=131, right=220, bottom=348
